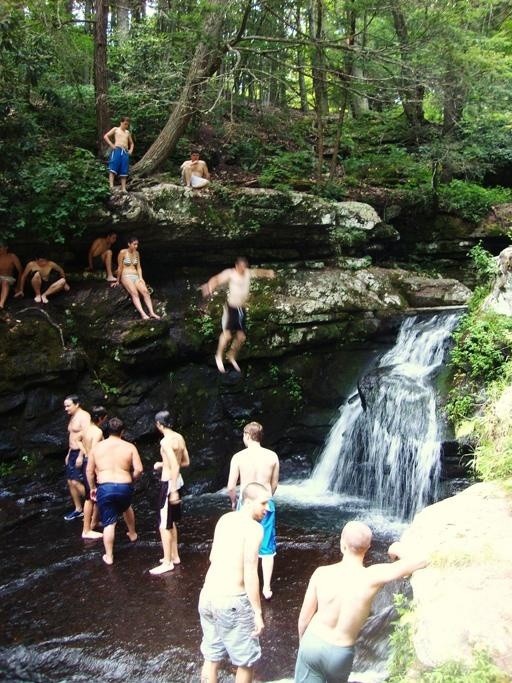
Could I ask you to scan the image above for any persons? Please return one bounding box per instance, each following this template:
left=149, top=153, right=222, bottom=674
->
left=87, top=231, right=118, bottom=282
left=295, top=520, right=430, bottom=683
left=110, top=237, right=160, bottom=320
left=64, top=394, right=91, bottom=521
left=197, top=481, right=269, bottom=682
left=103, top=117, right=133, bottom=193
left=180, top=150, right=210, bottom=188
left=148, top=411, right=190, bottom=576
left=12, top=252, right=70, bottom=304
left=74, top=405, right=107, bottom=539
left=227, top=421, right=279, bottom=599
left=0, top=239, right=22, bottom=309
left=201, top=256, right=274, bottom=373
left=86, top=418, right=143, bottom=565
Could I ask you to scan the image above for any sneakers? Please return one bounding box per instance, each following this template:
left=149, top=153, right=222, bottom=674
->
left=64, top=510, right=83, bottom=520
